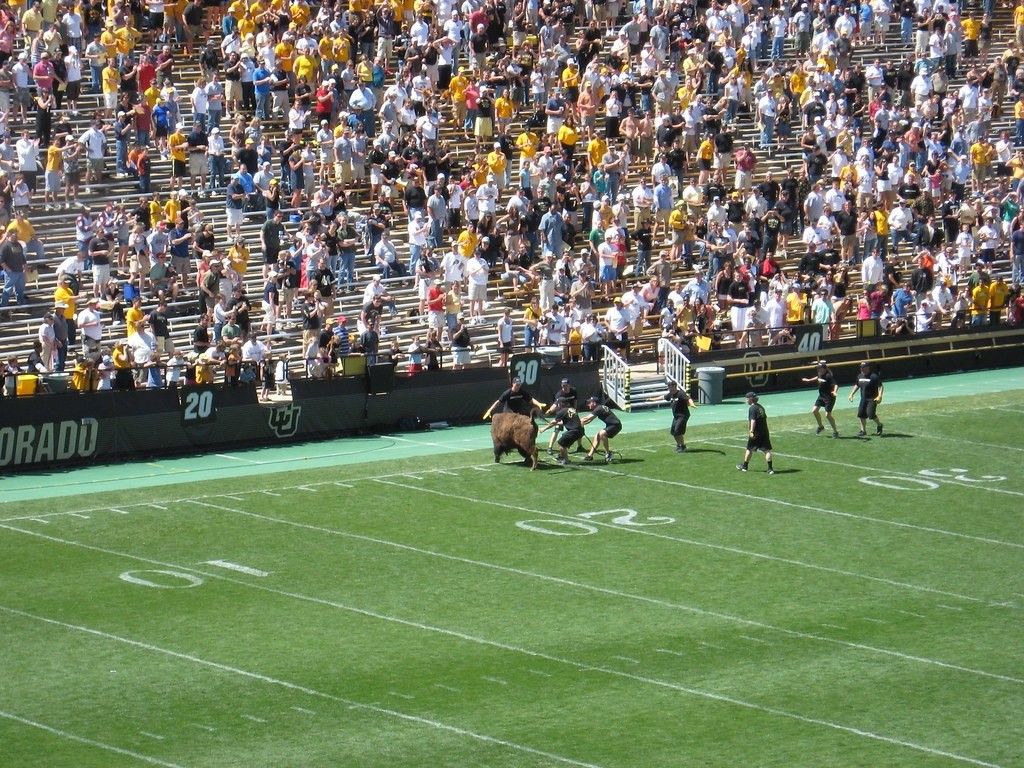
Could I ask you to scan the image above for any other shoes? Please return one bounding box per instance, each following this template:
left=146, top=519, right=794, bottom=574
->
left=577, top=447, right=586, bottom=451
left=584, top=456, right=592, bottom=460
left=768, top=470, right=774, bottom=474
left=816, top=426, right=824, bottom=435
left=736, top=464, right=747, bottom=472
left=554, top=456, right=570, bottom=464
left=676, top=445, right=686, bottom=451
left=832, top=432, right=837, bottom=438
left=606, top=453, right=612, bottom=461
left=547, top=449, right=553, bottom=455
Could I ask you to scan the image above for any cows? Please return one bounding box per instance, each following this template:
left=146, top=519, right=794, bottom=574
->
left=492, top=407, right=539, bottom=472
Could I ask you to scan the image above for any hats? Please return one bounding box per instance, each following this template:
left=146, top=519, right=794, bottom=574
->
left=665, top=381, right=677, bottom=386
left=2, top=0, right=1022, bottom=369
left=561, top=378, right=568, bottom=385
left=746, top=392, right=757, bottom=398
left=555, top=397, right=566, bottom=403
left=587, top=396, right=599, bottom=402
left=512, top=377, right=522, bottom=384
left=858, top=361, right=871, bottom=367
left=815, top=362, right=826, bottom=369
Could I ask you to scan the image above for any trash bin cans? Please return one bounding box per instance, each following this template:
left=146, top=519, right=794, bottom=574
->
left=695, top=366, right=725, bottom=404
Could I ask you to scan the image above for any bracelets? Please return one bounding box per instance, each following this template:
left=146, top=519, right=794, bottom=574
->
left=750, top=431, right=754, bottom=433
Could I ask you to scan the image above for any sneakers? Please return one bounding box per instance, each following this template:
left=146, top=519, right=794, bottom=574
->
left=877, top=424, right=883, bottom=435
left=858, top=431, right=865, bottom=435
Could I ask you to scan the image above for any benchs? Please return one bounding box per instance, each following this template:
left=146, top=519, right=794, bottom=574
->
left=1, top=1, right=1024, bottom=364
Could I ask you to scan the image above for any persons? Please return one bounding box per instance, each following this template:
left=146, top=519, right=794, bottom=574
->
left=545, top=378, right=578, bottom=454
left=801, top=362, right=838, bottom=439
left=849, top=362, right=883, bottom=436
left=482, top=377, right=546, bottom=420
left=0, top=0, right=1024, bottom=396
left=647, top=381, right=698, bottom=453
left=539, top=397, right=585, bottom=464
left=735, top=392, right=774, bottom=475
left=580, top=397, right=622, bottom=462
